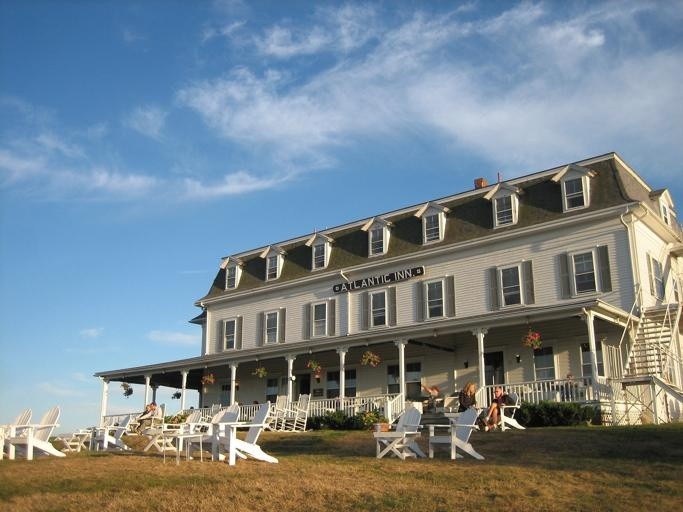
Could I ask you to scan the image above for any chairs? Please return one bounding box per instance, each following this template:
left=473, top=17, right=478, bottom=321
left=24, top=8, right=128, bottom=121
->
left=263, top=393, right=311, bottom=432
left=137, top=400, right=281, bottom=466
left=54, top=416, right=140, bottom=451
left=371, top=390, right=525, bottom=463
left=1, top=406, right=66, bottom=460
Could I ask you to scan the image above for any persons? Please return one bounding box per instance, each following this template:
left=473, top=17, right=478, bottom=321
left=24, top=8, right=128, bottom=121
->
left=139, top=404, right=151, bottom=425
left=457, top=382, right=477, bottom=415
left=418, top=382, right=440, bottom=412
left=136, top=401, right=162, bottom=433
left=480, top=385, right=515, bottom=432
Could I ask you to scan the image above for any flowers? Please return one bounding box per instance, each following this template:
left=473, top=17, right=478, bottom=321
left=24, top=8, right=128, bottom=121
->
left=306, top=361, right=322, bottom=378
left=519, top=322, right=542, bottom=351
left=200, top=372, right=218, bottom=385
left=360, top=350, right=383, bottom=368
